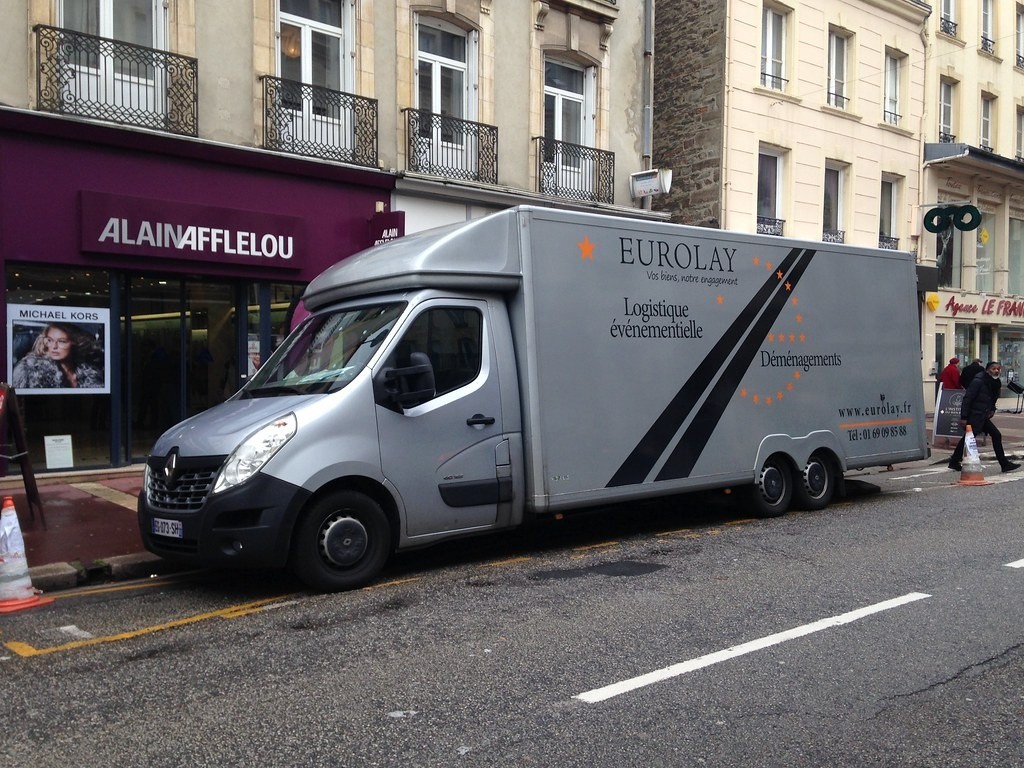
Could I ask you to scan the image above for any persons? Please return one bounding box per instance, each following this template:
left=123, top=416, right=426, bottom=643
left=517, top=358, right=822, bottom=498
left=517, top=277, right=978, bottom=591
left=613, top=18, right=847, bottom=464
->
left=940, top=358, right=964, bottom=389
left=960, top=359, right=984, bottom=390
left=948, top=362, right=1021, bottom=472
left=13, top=322, right=105, bottom=388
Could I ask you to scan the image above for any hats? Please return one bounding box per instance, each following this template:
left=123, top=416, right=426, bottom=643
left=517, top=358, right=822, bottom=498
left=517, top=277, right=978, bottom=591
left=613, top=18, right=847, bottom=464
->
left=985, top=361, right=996, bottom=370
left=972, top=359, right=983, bottom=363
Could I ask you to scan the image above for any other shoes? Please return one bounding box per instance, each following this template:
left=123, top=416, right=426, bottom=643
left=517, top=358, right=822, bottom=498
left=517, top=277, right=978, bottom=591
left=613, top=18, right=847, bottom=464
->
left=948, top=459, right=963, bottom=472
left=1001, top=461, right=1022, bottom=472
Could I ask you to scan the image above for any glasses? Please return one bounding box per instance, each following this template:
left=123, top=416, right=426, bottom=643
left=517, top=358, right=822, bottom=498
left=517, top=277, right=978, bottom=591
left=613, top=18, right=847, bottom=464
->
left=42, top=336, right=74, bottom=349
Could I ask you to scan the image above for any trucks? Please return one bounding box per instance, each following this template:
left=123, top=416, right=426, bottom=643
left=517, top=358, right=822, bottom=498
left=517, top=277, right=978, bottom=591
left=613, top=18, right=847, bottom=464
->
left=137, top=204, right=930, bottom=586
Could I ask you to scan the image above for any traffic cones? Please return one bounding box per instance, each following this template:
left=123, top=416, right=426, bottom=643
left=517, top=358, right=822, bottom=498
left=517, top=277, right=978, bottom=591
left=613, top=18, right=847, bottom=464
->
left=0, top=496, right=53, bottom=616
left=950, top=423, right=997, bottom=486
